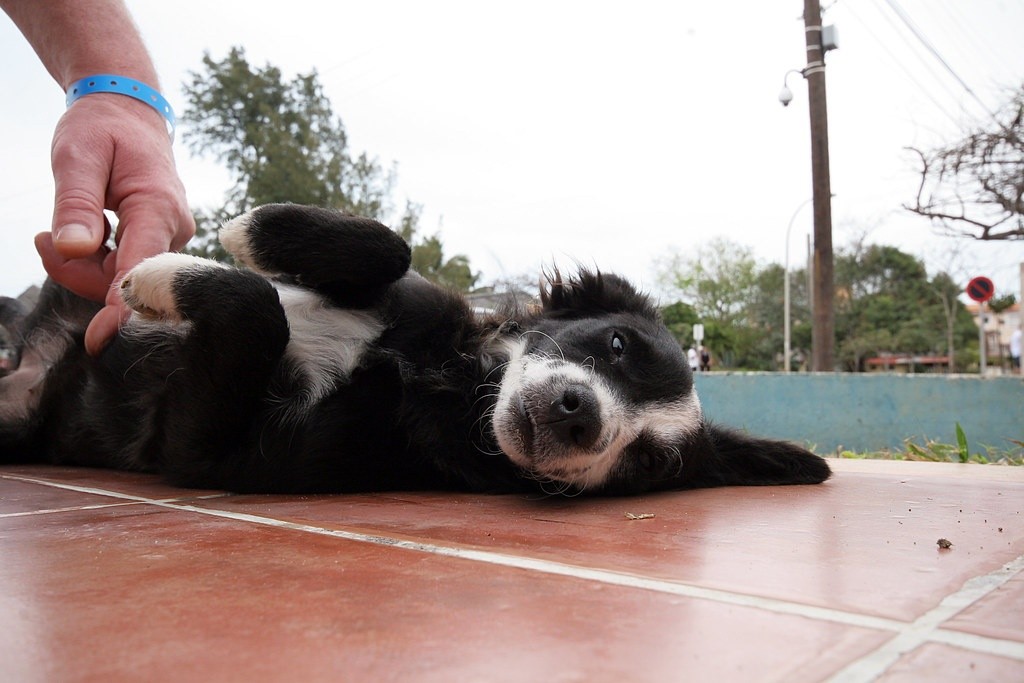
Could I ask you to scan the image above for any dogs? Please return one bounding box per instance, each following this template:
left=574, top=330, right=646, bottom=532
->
left=0, top=203, right=835, bottom=502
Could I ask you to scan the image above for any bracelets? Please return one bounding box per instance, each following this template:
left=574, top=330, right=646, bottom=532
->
left=66, top=74, right=178, bottom=146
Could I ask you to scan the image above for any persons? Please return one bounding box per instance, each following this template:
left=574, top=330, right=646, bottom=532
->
left=687, top=343, right=711, bottom=372
left=0, top=0, right=197, bottom=358
left=1010, top=325, right=1022, bottom=374
